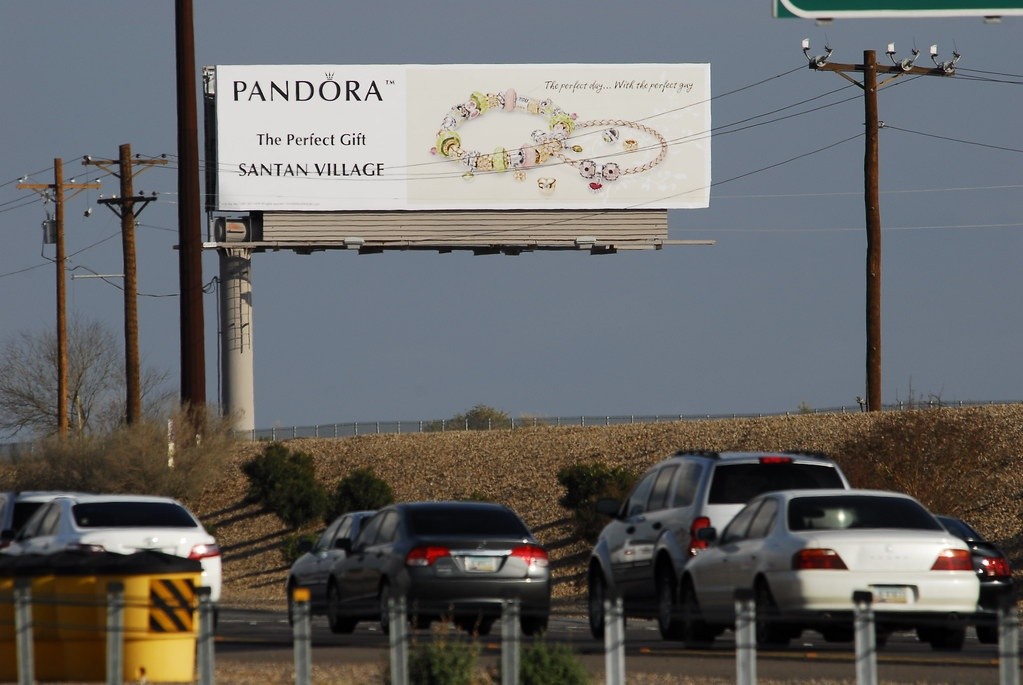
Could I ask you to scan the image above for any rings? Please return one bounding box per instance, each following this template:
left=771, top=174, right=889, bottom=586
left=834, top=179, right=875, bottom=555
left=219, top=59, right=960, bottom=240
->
left=536, top=178, right=556, bottom=195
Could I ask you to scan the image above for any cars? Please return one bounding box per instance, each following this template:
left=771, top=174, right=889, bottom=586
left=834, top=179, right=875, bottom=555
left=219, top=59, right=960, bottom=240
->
left=680, top=491, right=1018, bottom=650
left=0, top=489, right=224, bottom=602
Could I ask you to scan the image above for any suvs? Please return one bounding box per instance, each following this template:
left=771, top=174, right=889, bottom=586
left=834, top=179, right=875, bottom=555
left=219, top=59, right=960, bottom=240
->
left=283, top=502, right=554, bottom=637
left=588, top=447, right=851, bottom=645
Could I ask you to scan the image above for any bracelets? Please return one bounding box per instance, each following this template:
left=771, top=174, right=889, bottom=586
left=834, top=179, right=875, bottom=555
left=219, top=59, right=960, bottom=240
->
left=430, top=89, right=583, bottom=181
left=550, top=119, right=667, bottom=189
